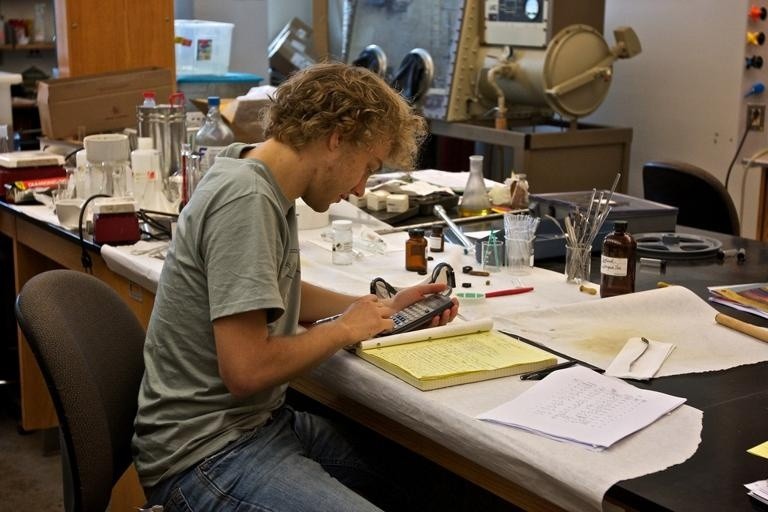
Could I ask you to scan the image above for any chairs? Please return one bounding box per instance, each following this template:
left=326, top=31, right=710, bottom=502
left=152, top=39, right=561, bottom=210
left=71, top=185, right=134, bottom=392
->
left=13, top=269, right=168, bottom=511
left=641, top=159, right=741, bottom=236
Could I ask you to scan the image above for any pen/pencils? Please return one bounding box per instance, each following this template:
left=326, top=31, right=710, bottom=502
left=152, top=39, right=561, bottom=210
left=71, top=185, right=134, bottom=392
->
left=486, top=287, right=534, bottom=297
left=312, top=314, right=341, bottom=326
left=484, top=229, right=498, bottom=268
left=520, top=361, right=577, bottom=380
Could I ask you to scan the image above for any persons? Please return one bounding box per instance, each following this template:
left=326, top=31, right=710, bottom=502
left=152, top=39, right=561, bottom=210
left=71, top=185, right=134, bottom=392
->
left=125, top=53, right=464, bottom=511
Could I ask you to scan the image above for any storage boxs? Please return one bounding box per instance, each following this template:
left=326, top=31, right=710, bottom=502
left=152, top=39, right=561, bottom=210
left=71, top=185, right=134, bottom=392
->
left=172, top=18, right=236, bottom=76
left=267, top=17, right=315, bottom=74
left=36, top=66, right=173, bottom=140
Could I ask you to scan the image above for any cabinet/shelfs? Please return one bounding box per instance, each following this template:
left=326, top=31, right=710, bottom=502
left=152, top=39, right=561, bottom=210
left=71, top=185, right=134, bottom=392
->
left=0, top=1, right=177, bottom=153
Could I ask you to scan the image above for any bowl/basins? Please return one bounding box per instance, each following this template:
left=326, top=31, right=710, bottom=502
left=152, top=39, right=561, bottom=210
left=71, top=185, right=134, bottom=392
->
left=54, top=197, right=91, bottom=229
left=83, top=133, right=131, bottom=164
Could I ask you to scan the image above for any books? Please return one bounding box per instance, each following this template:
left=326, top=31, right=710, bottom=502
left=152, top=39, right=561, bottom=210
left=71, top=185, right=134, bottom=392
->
left=0, top=152, right=72, bottom=205
left=704, top=279, right=768, bottom=342
left=343, top=313, right=561, bottom=394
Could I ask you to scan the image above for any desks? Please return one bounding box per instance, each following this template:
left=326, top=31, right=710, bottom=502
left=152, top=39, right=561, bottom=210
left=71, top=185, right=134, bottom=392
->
left=177, top=72, right=264, bottom=113
left=412, top=114, right=632, bottom=204
left=0, top=132, right=768, bottom=510
left=741, top=146, right=768, bottom=249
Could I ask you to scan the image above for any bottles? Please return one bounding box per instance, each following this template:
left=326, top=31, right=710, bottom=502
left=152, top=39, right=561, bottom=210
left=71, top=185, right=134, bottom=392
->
left=331, top=219, right=354, bottom=265
left=598, top=220, right=636, bottom=300
left=130, top=134, right=163, bottom=209
left=510, top=172, right=530, bottom=210
left=139, top=90, right=159, bottom=106
left=404, top=228, right=428, bottom=273
left=429, top=225, right=446, bottom=253
left=460, top=154, right=490, bottom=217
left=194, top=95, right=236, bottom=180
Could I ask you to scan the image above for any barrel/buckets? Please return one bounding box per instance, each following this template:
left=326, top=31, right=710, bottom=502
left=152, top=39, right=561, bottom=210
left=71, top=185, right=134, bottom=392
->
left=135, top=92, right=188, bottom=180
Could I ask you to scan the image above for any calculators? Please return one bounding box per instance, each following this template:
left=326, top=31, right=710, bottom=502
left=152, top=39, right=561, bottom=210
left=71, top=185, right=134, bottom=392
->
left=379, top=293, right=454, bottom=335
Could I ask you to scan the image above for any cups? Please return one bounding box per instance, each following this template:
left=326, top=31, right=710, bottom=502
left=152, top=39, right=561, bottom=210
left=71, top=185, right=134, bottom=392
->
left=503, top=234, right=536, bottom=276
left=135, top=104, right=188, bottom=176
left=481, top=239, right=503, bottom=274
left=564, top=242, right=592, bottom=284
